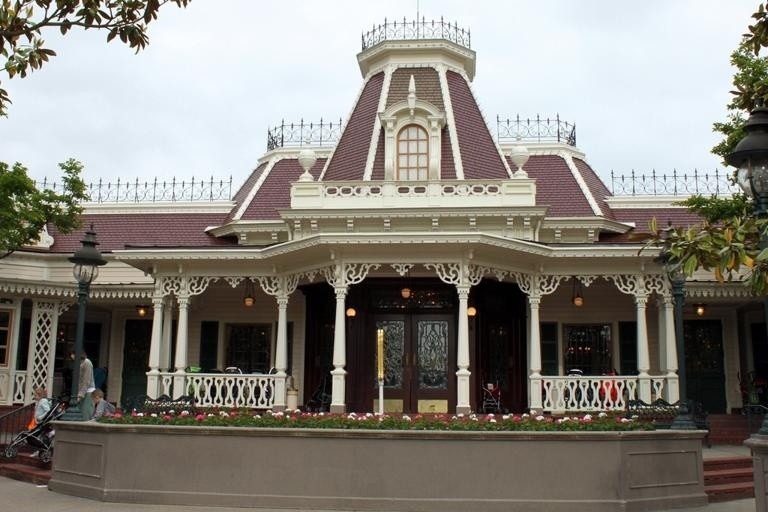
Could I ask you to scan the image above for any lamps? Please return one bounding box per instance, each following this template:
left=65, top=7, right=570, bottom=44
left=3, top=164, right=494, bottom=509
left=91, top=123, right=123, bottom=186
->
left=467, top=307, right=477, bottom=316
left=242, top=281, right=257, bottom=307
left=138, top=299, right=145, bottom=316
left=346, top=290, right=356, bottom=317
left=697, top=306, right=703, bottom=316
left=401, top=280, right=410, bottom=299
left=572, top=281, right=584, bottom=308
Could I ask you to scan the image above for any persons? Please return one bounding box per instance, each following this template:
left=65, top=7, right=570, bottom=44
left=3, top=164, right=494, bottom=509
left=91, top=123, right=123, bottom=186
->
left=89, top=389, right=114, bottom=422
left=70, top=348, right=97, bottom=421
left=28, top=386, right=51, bottom=457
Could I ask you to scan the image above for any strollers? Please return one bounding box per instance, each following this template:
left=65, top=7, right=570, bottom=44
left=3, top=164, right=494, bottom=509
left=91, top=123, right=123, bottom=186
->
left=4, top=400, right=69, bottom=464
left=478, top=367, right=509, bottom=414
left=737, top=371, right=767, bottom=415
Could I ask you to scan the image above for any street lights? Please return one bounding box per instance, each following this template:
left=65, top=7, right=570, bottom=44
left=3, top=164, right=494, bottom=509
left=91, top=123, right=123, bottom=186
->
left=655, top=222, right=692, bottom=415
left=59, top=221, right=108, bottom=407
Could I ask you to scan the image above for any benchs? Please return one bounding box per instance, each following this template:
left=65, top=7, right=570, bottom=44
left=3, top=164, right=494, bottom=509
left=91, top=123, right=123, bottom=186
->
left=626, top=398, right=711, bottom=448
left=123, top=392, right=194, bottom=414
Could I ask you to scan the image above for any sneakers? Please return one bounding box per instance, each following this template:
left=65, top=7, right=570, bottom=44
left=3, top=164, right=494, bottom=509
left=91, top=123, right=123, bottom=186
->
left=29, top=450, right=39, bottom=457
